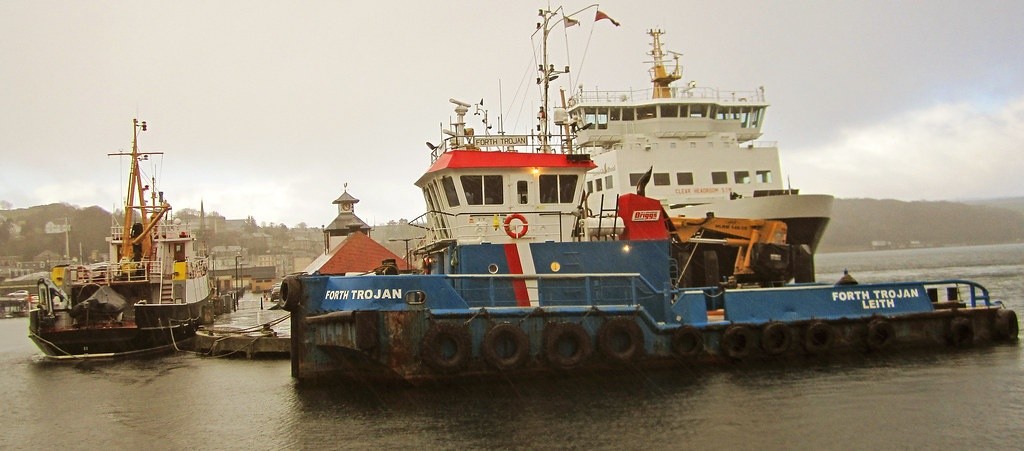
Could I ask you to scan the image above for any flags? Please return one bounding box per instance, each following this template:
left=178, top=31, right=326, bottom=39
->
left=596, top=11, right=619, bottom=27
left=564, top=16, right=580, bottom=28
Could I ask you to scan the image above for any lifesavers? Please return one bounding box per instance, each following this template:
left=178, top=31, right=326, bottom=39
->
left=990, top=308, right=1019, bottom=344
left=946, top=316, right=975, bottom=351
left=672, top=325, right=704, bottom=362
left=543, top=322, right=594, bottom=373
left=760, top=322, right=792, bottom=356
left=503, top=214, right=528, bottom=239
left=482, top=323, right=530, bottom=372
left=597, top=318, right=642, bottom=366
left=866, top=318, right=896, bottom=353
left=279, top=275, right=301, bottom=312
left=804, top=322, right=835, bottom=356
left=719, top=325, right=755, bottom=362
left=419, top=321, right=473, bottom=378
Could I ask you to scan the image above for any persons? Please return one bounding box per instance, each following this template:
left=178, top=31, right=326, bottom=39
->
left=571, top=206, right=585, bottom=242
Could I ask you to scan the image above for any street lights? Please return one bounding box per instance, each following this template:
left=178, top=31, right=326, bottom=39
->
left=235, top=255, right=245, bottom=307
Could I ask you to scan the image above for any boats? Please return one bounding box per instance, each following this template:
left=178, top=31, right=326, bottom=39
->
left=29, top=115, right=220, bottom=364
left=277, top=0, right=1018, bottom=392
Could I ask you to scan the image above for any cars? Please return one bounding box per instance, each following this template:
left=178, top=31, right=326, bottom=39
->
left=271, top=286, right=282, bottom=301
left=5, top=290, right=40, bottom=304
